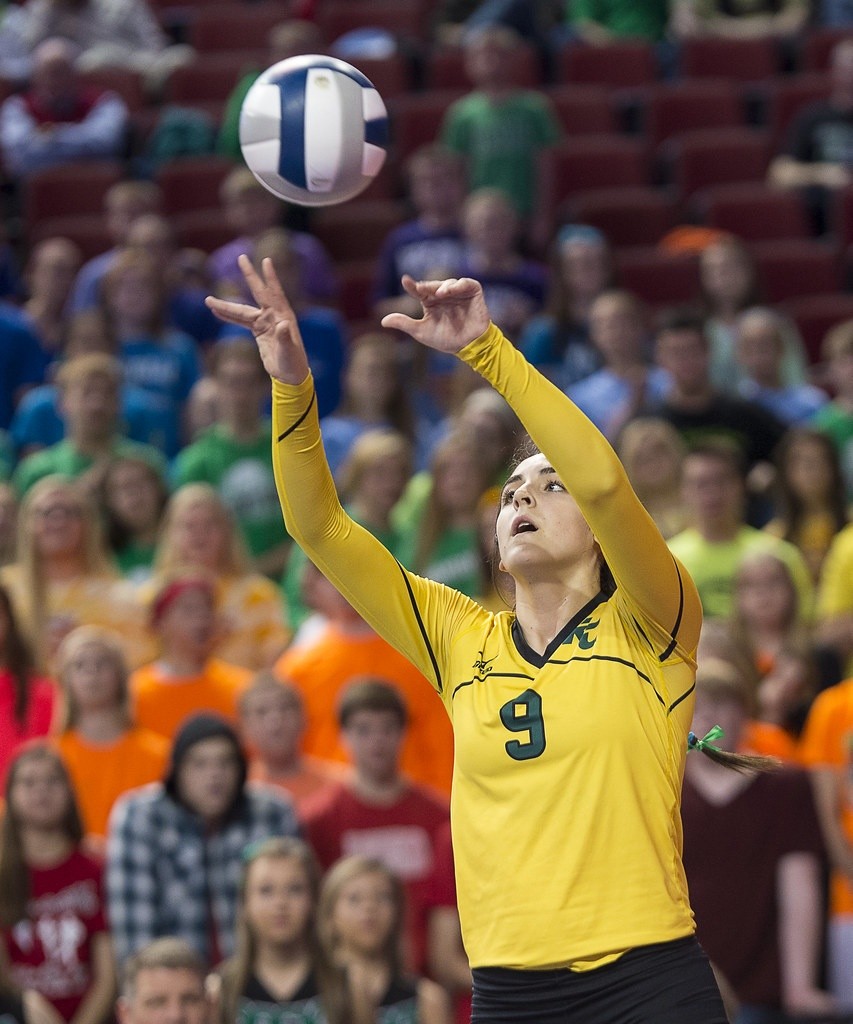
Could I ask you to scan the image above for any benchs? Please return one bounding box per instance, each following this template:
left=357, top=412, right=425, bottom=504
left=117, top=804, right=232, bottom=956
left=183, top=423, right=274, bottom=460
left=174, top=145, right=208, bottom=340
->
left=0, top=0, right=853, bottom=375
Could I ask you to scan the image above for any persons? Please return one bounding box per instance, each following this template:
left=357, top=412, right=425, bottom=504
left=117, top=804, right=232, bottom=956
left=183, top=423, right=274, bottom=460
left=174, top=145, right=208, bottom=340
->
left=0, top=0, right=853, bottom=1024
left=204, top=249, right=725, bottom=1023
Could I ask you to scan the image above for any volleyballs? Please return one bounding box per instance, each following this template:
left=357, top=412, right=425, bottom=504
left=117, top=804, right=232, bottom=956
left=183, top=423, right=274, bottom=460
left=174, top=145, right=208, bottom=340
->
left=238, top=54, right=391, bottom=207
